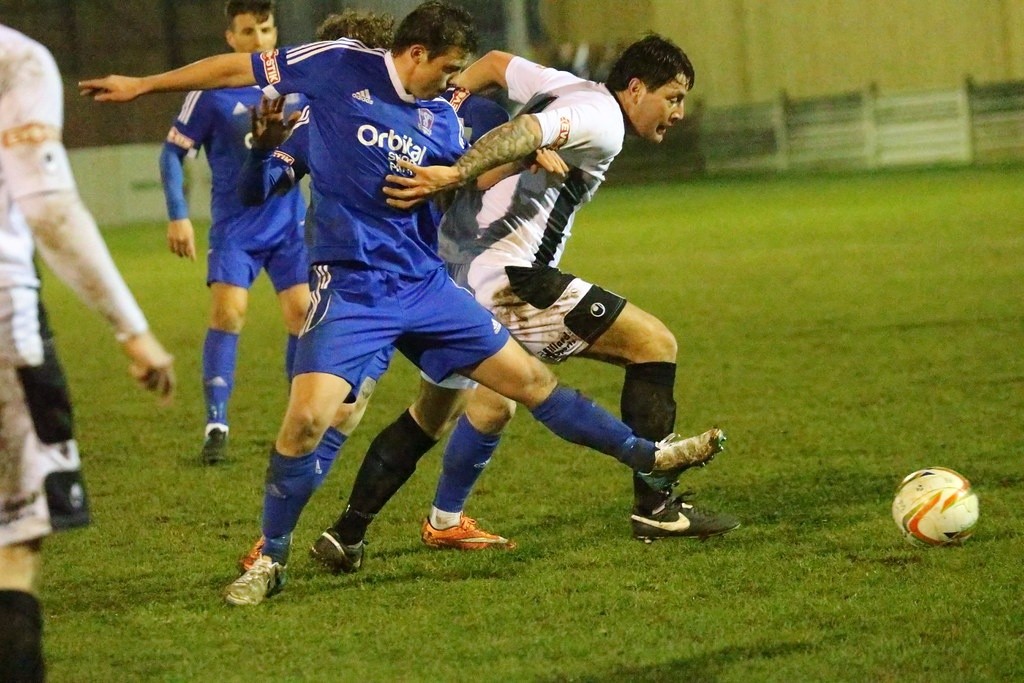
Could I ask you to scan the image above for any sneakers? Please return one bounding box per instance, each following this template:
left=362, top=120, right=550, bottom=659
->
left=242, top=537, right=266, bottom=570
left=638, top=428, right=726, bottom=498
left=422, top=512, right=517, bottom=553
left=632, top=491, right=740, bottom=541
left=201, top=426, right=227, bottom=461
left=225, top=557, right=288, bottom=608
left=309, top=528, right=369, bottom=572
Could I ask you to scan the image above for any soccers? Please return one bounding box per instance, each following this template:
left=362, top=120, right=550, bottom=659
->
left=891, top=466, right=981, bottom=549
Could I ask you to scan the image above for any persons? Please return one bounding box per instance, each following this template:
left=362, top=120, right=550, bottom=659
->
left=78, top=0, right=742, bottom=605
left=16, top=264, right=91, bottom=525
left=161, top=0, right=309, bottom=462
left=1, top=21, right=173, bottom=683
